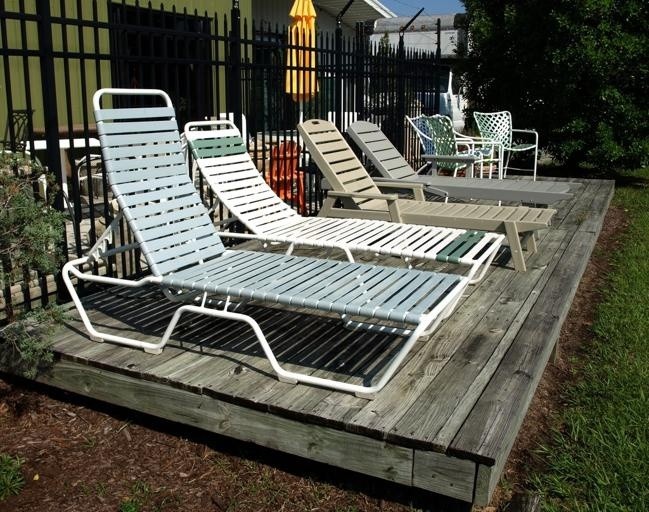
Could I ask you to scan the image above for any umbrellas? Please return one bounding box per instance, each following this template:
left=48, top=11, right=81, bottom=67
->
left=282, top=0, right=318, bottom=128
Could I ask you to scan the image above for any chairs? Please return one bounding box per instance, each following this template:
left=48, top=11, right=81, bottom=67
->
left=406, top=113, right=479, bottom=176
left=180, top=120, right=506, bottom=287
left=425, top=115, right=503, bottom=180
left=61, top=89, right=468, bottom=395
left=473, top=111, right=539, bottom=181
left=347, top=123, right=579, bottom=211
left=297, top=118, right=556, bottom=271
